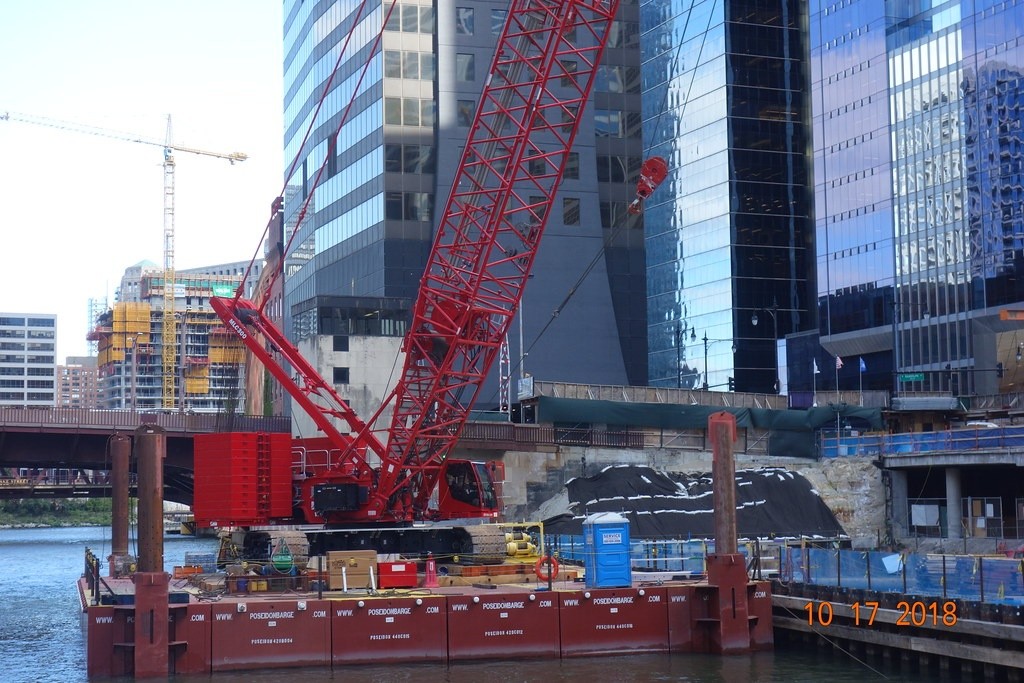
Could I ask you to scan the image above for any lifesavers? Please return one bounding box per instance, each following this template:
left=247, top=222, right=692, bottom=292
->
left=535, top=556, right=558, bottom=581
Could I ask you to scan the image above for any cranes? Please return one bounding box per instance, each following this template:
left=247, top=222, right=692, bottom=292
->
left=0, top=110, right=250, bottom=411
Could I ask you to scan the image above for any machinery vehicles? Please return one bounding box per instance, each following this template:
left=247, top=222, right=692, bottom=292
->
left=192, top=0, right=668, bottom=573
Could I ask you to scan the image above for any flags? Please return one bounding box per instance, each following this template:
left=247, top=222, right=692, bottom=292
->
left=860, top=358, right=866, bottom=372
left=836, top=356, right=844, bottom=369
left=814, top=358, right=821, bottom=374
